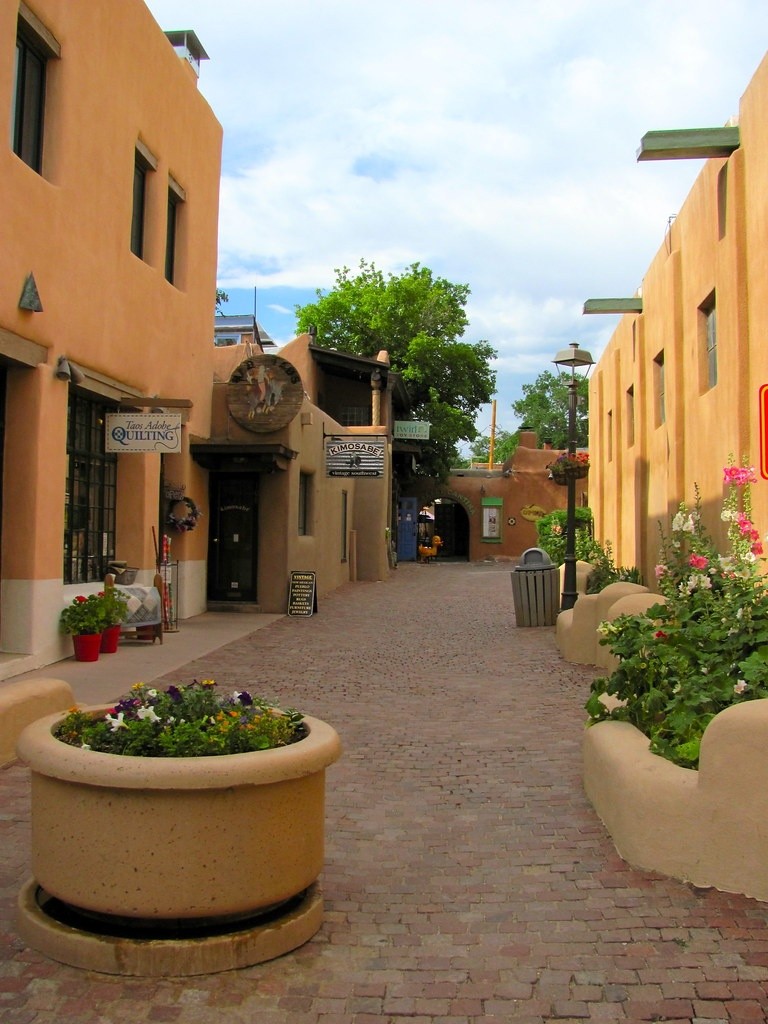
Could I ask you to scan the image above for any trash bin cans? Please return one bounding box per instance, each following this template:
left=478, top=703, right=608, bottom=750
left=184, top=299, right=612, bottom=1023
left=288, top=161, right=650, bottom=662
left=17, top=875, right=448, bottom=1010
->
left=510, top=548, right=560, bottom=627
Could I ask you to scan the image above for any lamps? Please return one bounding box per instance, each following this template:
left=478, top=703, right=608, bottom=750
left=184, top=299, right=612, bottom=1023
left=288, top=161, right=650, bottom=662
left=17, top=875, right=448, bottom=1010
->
left=57, top=355, right=85, bottom=385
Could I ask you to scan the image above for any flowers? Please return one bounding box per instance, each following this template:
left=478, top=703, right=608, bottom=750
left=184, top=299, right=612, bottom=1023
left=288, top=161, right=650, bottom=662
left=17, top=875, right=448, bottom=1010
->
left=61, top=675, right=306, bottom=751
left=168, top=511, right=197, bottom=534
left=59, top=596, right=105, bottom=634
left=545, top=452, right=568, bottom=471
left=94, top=588, right=132, bottom=625
left=569, top=452, right=589, bottom=466
left=584, top=453, right=768, bottom=773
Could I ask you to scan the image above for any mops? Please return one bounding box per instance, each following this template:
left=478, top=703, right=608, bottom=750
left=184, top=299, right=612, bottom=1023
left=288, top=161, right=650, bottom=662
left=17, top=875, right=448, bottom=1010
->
left=150, top=526, right=164, bottom=599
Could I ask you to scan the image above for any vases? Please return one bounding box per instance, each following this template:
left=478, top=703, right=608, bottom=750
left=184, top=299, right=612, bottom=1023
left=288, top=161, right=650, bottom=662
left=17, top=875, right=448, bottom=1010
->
left=100, top=626, right=122, bottom=656
left=570, top=467, right=589, bottom=479
left=11, top=700, right=341, bottom=976
left=71, top=635, right=103, bottom=662
left=552, top=469, right=566, bottom=485
